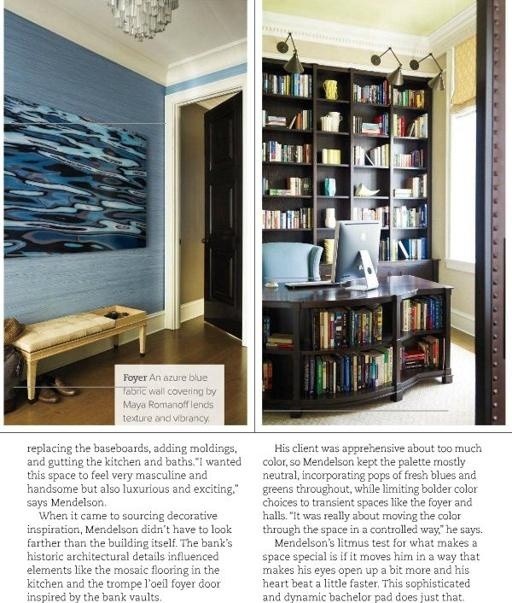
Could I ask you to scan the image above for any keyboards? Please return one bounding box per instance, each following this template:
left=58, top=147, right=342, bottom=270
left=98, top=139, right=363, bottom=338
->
left=285, top=280, right=352, bottom=290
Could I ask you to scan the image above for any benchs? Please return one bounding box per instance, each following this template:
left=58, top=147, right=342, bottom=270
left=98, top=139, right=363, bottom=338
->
left=7, top=303, right=150, bottom=405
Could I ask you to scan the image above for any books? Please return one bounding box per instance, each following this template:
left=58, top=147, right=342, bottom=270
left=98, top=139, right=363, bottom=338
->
left=261, top=68, right=445, bottom=402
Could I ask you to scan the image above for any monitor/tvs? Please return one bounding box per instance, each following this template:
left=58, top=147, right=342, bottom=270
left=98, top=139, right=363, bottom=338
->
left=331, top=220, right=381, bottom=291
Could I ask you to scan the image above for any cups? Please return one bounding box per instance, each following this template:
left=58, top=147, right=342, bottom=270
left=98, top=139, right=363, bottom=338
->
left=329, top=111, right=342, bottom=131
left=324, top=238, right=334, bottom=264
left=320, top=115, right=332, bottom=130
left=301, top=177, right=312, bottom=195
left=323, top=78, right=338, bottom=99
left=324, top=177, right=336, bottom=195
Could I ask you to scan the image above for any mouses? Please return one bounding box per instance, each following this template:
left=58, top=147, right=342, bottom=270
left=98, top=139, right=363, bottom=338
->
left=264, top=281, right=279, bottom=288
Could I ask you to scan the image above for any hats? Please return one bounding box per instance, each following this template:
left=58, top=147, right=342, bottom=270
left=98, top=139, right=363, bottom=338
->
left=4, top=318, right=27, bottom=343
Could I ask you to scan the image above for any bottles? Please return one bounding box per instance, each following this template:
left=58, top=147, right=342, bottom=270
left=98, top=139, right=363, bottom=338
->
left=325, top=207, right=336, bottom=227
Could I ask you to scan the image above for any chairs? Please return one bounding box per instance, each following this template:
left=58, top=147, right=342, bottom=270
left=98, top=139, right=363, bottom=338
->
left=262, top=241, right=325, bottom=285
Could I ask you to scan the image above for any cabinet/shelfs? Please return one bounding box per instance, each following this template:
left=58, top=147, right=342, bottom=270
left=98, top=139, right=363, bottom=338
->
left=261, top=59, right=439, bottom=284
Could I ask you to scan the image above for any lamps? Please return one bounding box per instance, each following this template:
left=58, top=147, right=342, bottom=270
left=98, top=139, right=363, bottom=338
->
left=276, top=31, right=305, bottom=75
left=104, top=0, right=179, bottom=44
left=371, top=45, right=448, bottom=92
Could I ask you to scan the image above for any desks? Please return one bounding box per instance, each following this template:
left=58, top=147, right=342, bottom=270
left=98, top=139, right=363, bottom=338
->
left=262, top=274, right=457, bottom=419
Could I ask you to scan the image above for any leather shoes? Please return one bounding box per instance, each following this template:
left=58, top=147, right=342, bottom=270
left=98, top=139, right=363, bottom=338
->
left=47, top=377, right=78, bottom=396
left=38, top=389, right=58, bottom=402
